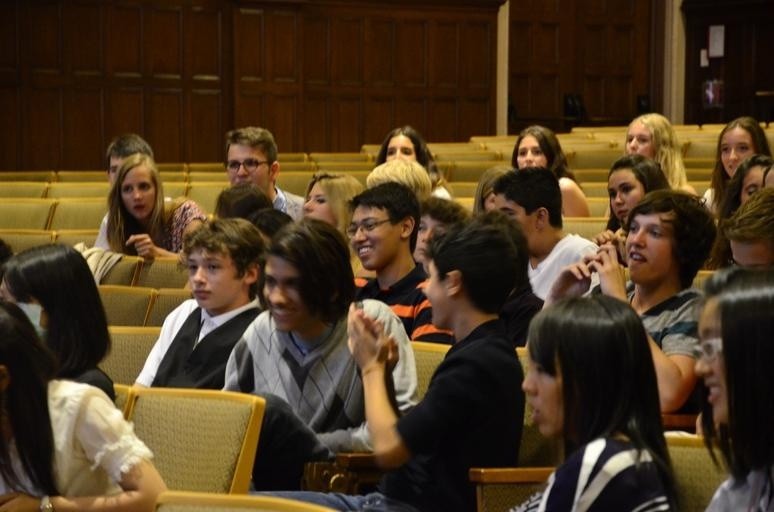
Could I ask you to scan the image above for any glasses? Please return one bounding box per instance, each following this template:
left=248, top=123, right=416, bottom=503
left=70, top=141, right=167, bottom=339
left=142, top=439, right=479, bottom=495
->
left=225, top=158, right=266, bottom=174
left=691, top=337, right=722, bottom=360
left=345, top=218, right=392, bottom=239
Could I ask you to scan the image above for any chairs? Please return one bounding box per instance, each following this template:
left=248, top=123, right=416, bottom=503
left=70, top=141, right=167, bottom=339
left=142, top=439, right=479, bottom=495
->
left=1, top=120, right=772, bottom=512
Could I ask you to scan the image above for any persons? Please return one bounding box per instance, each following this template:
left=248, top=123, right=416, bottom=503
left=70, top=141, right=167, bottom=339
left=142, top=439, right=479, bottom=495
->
left=94, top=133, right=207, bottom=258
left=1, top=240, right=170, bottom=510
left=132, top=113, right=774, bottom=510
